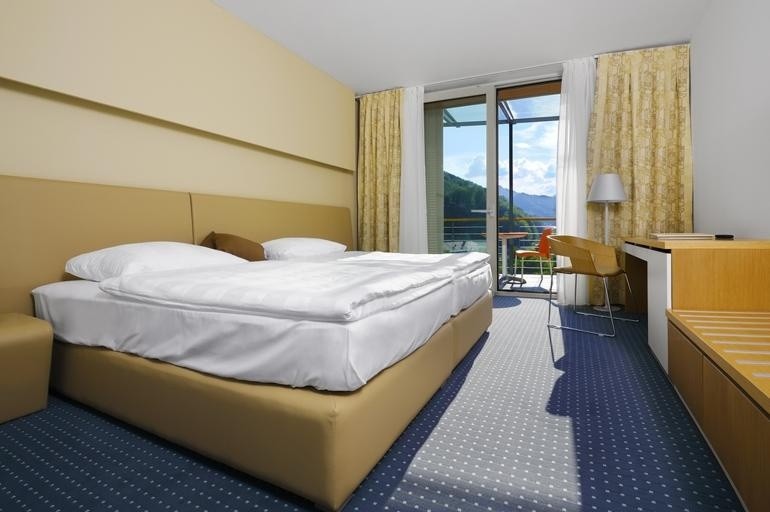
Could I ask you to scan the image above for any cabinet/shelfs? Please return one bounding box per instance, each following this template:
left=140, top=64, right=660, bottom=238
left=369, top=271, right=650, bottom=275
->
left=667, top=309, right=770, bottom=511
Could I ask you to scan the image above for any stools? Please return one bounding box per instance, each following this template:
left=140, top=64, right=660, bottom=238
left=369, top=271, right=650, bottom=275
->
left=0, top=313, right=54, bottom=422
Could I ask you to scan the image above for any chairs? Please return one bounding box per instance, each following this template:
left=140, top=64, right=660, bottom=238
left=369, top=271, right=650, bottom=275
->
left=546, top=234, right=640, bottom=337
left=513, top=227, right=553, bottom=285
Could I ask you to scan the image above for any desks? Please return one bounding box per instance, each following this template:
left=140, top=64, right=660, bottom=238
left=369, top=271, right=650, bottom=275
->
left=622, top=239, right=770, bottom=375
left=480, top=232, right=527, bottom=280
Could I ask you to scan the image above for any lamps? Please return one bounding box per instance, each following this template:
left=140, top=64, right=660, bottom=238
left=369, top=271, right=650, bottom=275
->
left=586, top=173, right=627, bottom=312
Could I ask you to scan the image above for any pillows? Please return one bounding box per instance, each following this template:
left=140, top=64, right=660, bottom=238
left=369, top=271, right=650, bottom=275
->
left=63, top=239, right=248, bottom=280
left=200, top=232, right=265, bottom=261
left=262, top=237, right=347, bottom=259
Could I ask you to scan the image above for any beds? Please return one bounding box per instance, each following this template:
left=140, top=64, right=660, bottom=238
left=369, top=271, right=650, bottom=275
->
left=0, top=176, right=493, bottom=511
